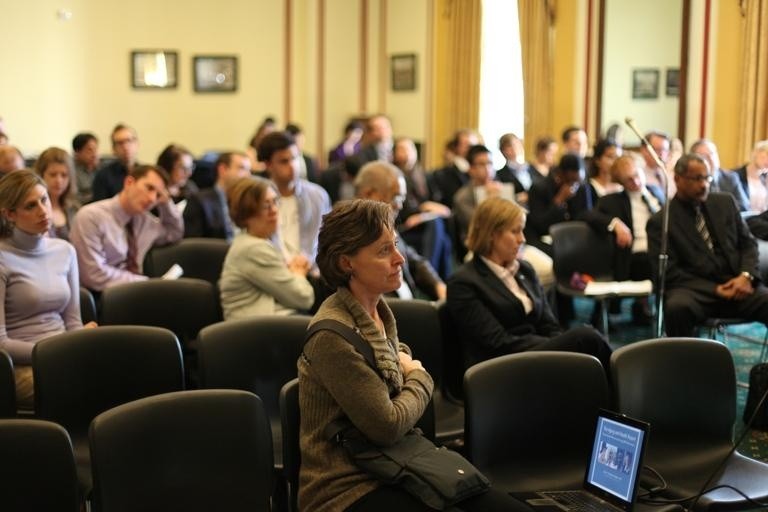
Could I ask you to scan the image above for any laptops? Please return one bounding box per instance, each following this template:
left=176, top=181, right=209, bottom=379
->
left=507, top=407, right=652, bottom=512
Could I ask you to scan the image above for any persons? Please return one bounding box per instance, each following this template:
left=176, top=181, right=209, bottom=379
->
left=295, top=199, right=493, bottom=512
left=0, top=115, right=768, bottom=399
left=597, top=442, right=632, bottom=475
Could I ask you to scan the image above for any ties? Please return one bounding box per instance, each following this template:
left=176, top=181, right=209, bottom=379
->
left=695, top=205, right=714, bottom=253
left=126, top=220, right=138, bottom=274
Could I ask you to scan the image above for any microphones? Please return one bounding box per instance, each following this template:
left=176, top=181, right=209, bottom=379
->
left=625, top=115, right=649, bottom=146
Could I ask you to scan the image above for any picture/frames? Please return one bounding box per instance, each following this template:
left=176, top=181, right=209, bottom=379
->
left=132, top=49, right=239, bottom=93
left=389, top=52, right=415, bottom=91
left=631, top=68, right=681, bottom=98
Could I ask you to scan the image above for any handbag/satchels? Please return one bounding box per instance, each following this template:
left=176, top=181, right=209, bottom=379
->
left=339, top=426, right=492, bottom=510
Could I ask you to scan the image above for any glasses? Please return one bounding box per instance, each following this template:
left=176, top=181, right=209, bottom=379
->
left=691, top=175, right=714, bottom=184
